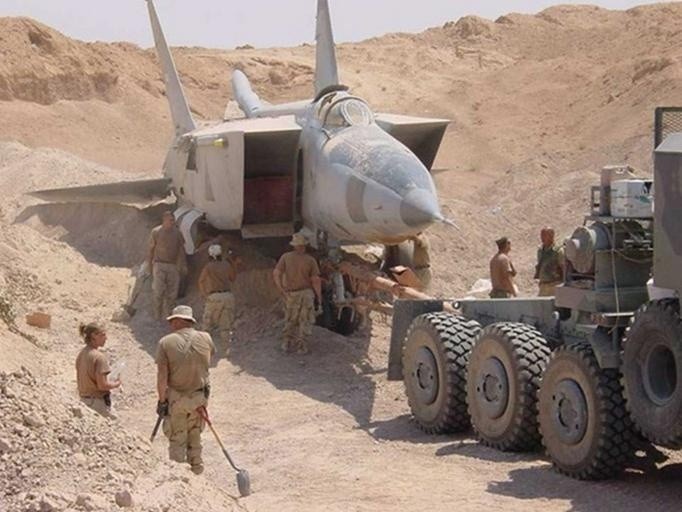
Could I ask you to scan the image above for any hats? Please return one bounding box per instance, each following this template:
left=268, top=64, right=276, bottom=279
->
left=165, top=304, right=197, bottom=324
left=289, top=232, right=308, bottom=247
left=497, top=236, right=512, bottom=245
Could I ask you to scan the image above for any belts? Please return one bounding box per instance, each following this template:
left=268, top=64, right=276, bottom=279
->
left=287, top=287, right=312, bottom=292
left=207, top=289, right=232, bottom=294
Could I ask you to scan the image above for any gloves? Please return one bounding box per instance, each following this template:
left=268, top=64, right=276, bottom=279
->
left=157, top=400, right=171, bottom=416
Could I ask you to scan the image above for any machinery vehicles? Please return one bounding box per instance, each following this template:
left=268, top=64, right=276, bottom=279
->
left=385, top=105, right=682, bottom=480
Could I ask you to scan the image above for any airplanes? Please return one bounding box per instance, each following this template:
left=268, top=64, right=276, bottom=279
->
left=147, top=0, right=453, bottom=335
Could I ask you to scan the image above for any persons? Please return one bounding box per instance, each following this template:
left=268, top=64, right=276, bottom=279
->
left=534, top=225, right=567, bottom=299
left=142, top=207, right=189, bottom=321
left=272, top=231, right=325, bottom=355
left=407, top=229, right=434, bottom=298
left=74, top=318, right=122, bottom=422
left=197, top=244, right=239, bottom=360
left=487, top=236, right=521, bottom=300
left=153, top=303, right=218, bottom=477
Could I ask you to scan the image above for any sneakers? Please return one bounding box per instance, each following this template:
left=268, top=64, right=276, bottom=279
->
left=279, top=340, right=310, bottom=355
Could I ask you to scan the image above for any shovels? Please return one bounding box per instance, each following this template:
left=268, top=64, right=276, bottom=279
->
left=121, top=272, right=151, bottom=316
left=196, top=405, right=250, bottom=496
